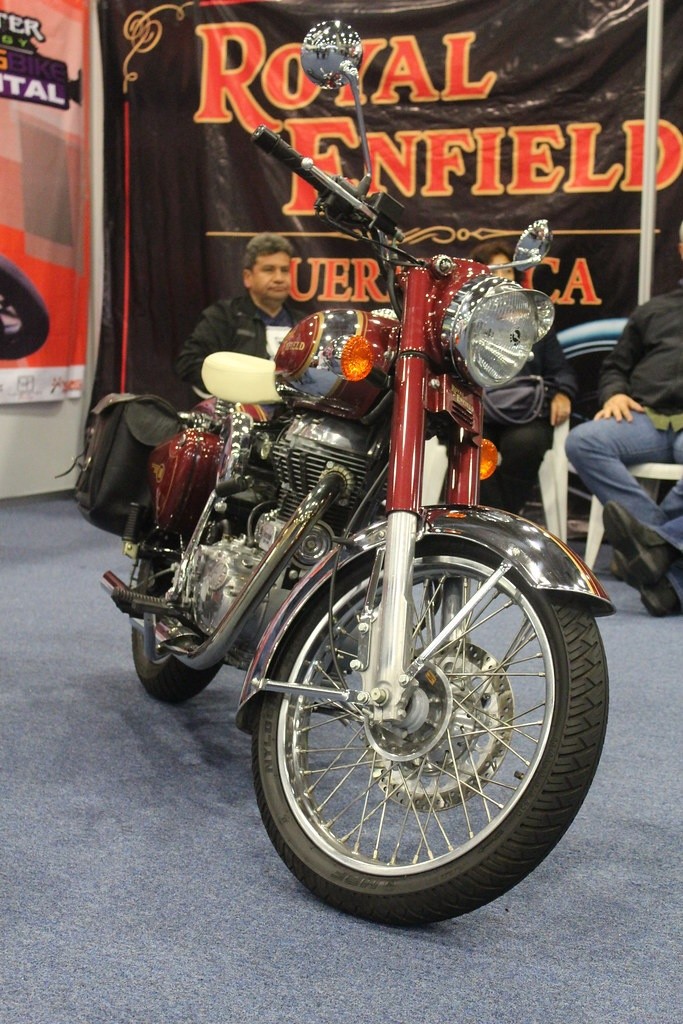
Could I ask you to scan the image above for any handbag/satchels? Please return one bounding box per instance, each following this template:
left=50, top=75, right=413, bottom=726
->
left=74, top=391, right=180, bottom=539
left=482, top=375, right=556, bottom=427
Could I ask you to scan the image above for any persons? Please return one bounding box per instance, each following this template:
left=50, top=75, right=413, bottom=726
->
left=452, top=241, right=578, bottom=517
left=178, top=233, right=310, bottom=419
left=565, top=218, right=683, bottom=617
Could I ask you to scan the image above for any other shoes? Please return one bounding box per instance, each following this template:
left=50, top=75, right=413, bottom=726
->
left=614, top=549, right=681, bottom=617
left=603, top=500, right=672, bottom=586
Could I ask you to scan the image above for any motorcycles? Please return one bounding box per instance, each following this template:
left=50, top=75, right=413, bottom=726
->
left=91, top=20, right=618, bottom=925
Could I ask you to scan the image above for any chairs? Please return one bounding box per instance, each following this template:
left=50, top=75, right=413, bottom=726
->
left=536, top=416, right=683, bottom=573
